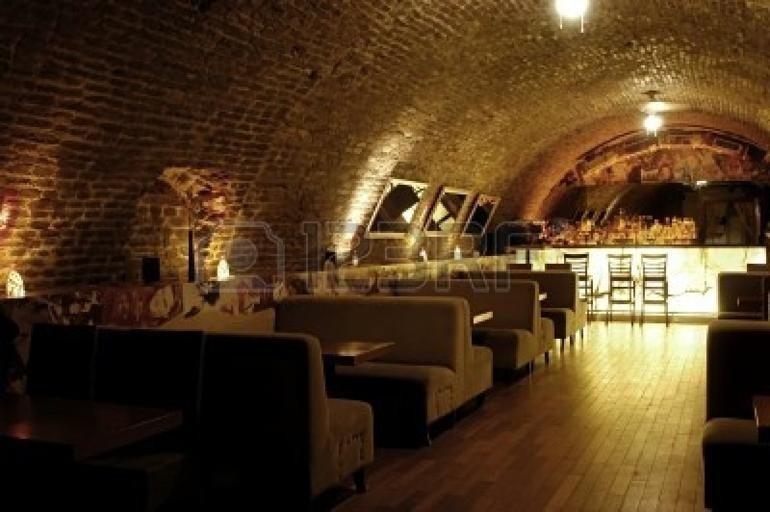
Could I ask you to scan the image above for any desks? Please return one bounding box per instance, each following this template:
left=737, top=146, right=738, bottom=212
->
left=1, top=398, right=184, bottom=512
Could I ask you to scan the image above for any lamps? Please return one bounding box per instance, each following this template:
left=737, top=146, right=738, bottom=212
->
left=555, top=0, right=590, bottom=34
left=641, top=90, right=663, bottom=137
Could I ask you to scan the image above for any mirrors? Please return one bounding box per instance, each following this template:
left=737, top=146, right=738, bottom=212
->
left=459, top=193, right=502, bottom=240
left=363, top=177, right=430, bottom=240
left=421, top=186, right=472, bottom=238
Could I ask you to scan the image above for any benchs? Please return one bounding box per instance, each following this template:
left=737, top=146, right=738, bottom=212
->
left=27, top=263, right=588, bottom=512
left=702, top=264, right=770, bottom=512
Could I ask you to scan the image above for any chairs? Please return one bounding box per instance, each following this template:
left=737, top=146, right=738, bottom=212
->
left=563, top=253, right=669, bottom=305
